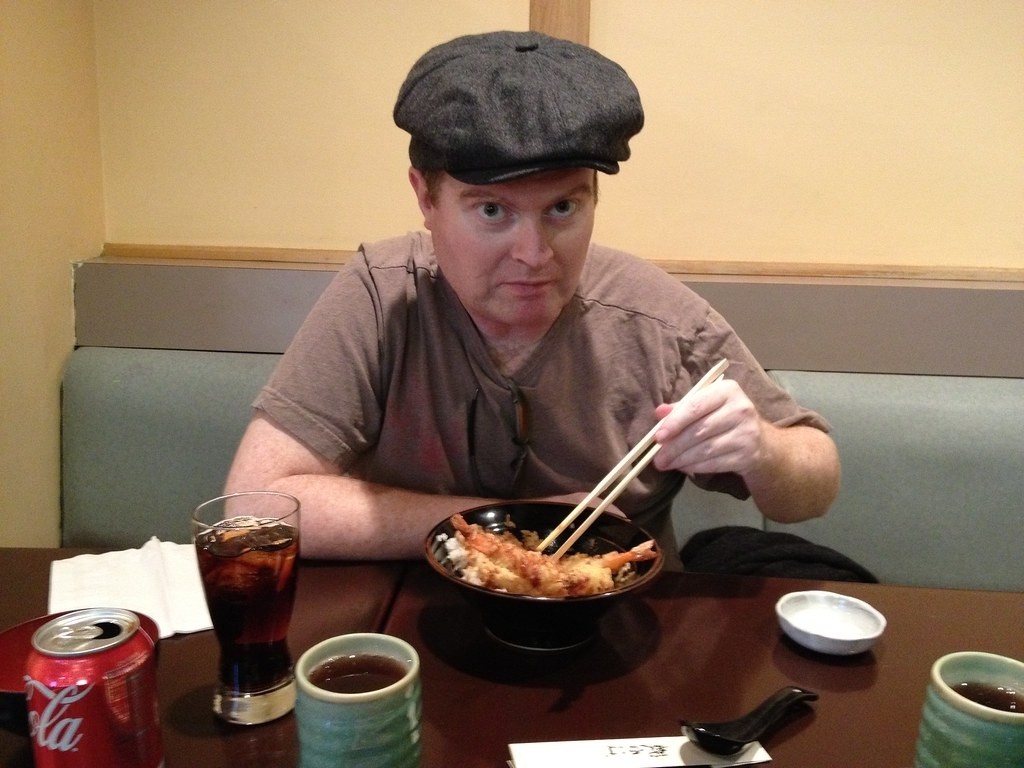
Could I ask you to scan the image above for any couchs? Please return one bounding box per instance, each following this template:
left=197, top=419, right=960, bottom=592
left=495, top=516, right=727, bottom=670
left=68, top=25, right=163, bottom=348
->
left=52, top=243, right=1023, bottom=589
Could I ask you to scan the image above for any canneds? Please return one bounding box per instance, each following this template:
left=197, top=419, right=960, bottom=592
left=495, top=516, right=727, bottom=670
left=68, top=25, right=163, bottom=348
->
left=23, top=606, right=168, bottom=768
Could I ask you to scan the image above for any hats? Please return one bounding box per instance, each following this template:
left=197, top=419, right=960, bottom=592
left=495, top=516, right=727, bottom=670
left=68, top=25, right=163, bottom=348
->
left=392, top=27, right=644, bottom=187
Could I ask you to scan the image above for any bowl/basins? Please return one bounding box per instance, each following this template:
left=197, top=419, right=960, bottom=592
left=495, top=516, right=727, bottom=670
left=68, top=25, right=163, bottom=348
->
left=0, top=609, right=159, bottom=736
left=425, top=499, right=664, bottom=651
left=776, top=589, right=887, bottom=655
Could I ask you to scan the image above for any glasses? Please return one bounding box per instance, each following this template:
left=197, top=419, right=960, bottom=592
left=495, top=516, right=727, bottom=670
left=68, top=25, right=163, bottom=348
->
left=467, top=374, right=529, bottom=500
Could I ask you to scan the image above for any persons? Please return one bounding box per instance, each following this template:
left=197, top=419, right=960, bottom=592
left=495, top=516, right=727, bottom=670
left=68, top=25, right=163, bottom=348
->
left=221, top=30, right=841, bottom=575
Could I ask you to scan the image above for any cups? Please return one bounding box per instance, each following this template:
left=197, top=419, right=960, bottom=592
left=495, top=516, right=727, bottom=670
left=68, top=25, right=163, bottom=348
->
left=295, top=633, right=422, bottom=768
left=913, top=653, right=1023, bottom=768
left=192, top=491, right=299, bottom=726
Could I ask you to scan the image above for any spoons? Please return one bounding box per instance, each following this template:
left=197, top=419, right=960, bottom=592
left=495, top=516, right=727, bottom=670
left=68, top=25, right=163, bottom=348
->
left=678, top=685, right=820, bottom=757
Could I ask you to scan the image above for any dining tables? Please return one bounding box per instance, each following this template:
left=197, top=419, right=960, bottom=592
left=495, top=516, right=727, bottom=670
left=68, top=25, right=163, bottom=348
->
left=2, top=541, right=1024, bottom=768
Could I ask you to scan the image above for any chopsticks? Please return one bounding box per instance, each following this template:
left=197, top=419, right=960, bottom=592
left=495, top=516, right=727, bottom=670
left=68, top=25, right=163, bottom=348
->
left=537, top=357, right=730, bottom=566
